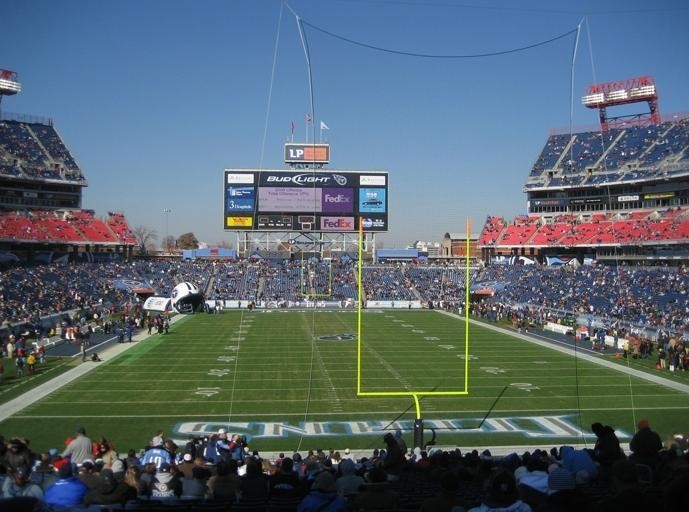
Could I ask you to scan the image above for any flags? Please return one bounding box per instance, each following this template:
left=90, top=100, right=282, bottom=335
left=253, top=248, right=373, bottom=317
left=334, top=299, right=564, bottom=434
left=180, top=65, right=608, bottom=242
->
left=304, top=114, right=311, bottom=121
left=292, top=122, right=295, bottom=134
left=321, top=121, right=330, bottom=130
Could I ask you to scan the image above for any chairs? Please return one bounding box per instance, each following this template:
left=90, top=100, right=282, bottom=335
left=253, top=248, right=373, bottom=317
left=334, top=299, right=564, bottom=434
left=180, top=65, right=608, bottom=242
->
left=0, top=455, right=689, bottom=512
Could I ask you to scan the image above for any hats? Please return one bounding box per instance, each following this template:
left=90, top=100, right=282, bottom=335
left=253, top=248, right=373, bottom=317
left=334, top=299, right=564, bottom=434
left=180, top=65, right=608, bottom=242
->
left=52, top=457, right=123, bottom=494
left=281, top=447, right=325, bottom=472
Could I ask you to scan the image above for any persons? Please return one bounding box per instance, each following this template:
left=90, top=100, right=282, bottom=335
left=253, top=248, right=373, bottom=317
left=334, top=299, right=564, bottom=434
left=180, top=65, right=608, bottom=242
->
left=476, top=119, right=689, bottom=371
left=1, top=417, right=689, bottom=511
left=215, top=257, right=476, bottom=315
left=1, top=117, right=215, bottom=375
